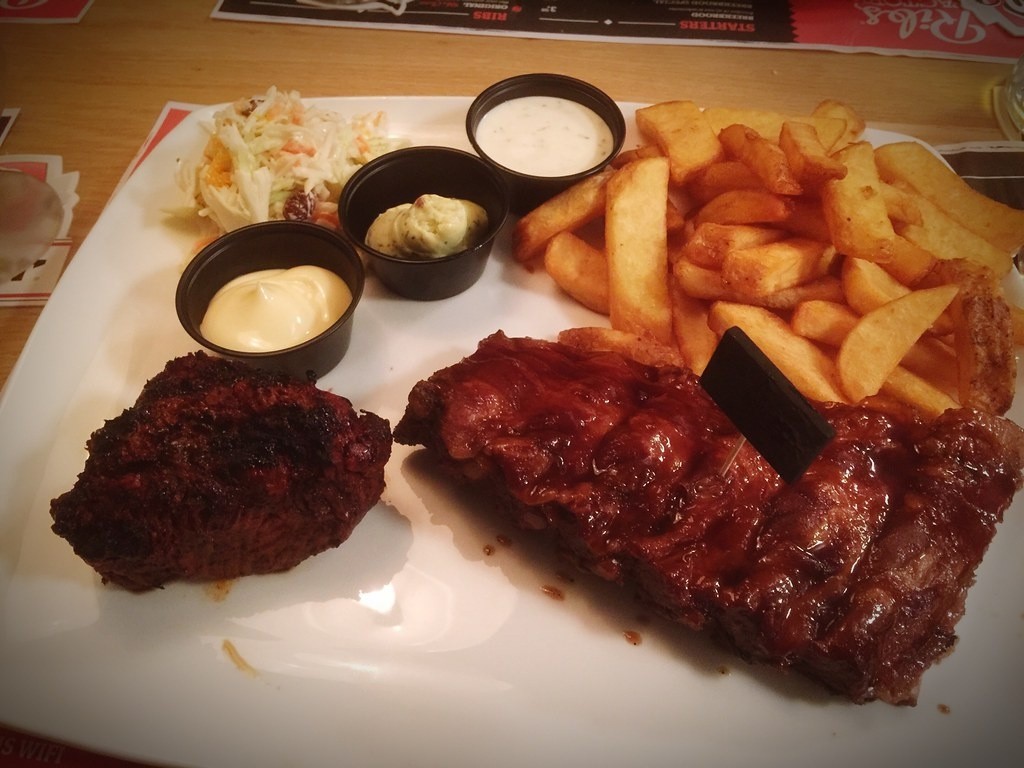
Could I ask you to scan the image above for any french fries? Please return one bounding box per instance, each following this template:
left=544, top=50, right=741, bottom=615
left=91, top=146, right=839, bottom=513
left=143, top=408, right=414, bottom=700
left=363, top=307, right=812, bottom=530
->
left=514, top=100, right=1024, bottom=414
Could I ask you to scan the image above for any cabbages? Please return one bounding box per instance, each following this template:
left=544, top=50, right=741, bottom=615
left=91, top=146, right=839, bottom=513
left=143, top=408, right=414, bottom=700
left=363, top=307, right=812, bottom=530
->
left=193, top=90, right=409, bottom=235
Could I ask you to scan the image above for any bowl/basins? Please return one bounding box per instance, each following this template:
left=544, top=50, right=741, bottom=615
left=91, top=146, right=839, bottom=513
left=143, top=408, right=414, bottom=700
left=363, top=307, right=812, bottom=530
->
left=175, top=220, right=366, bottom=386
left=338, top=145, right=509, bottom=300
left=466, top=72, right=626, bottom=217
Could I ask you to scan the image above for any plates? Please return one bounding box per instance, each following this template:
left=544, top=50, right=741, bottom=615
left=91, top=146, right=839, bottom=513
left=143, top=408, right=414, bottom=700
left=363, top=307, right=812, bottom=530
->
left=0, top=95, right=1024, bottom=768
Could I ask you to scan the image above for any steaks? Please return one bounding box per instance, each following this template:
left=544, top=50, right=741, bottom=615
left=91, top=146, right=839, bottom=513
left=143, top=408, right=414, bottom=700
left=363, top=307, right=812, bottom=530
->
left=392, top=331, right=1023, bottom=706
